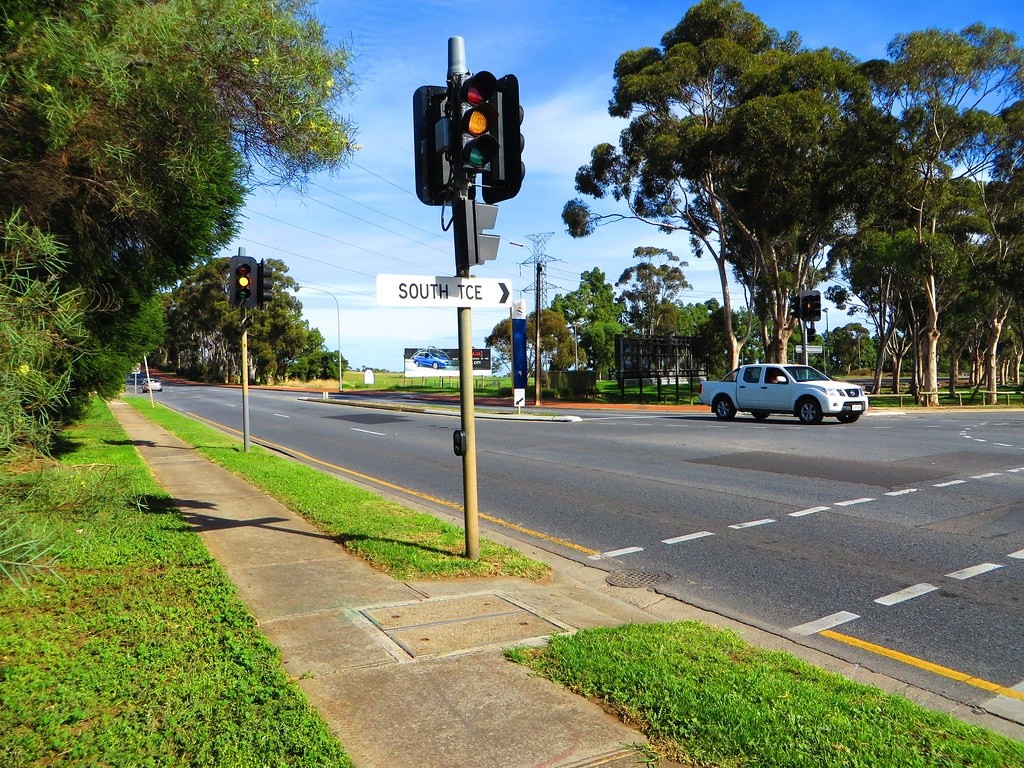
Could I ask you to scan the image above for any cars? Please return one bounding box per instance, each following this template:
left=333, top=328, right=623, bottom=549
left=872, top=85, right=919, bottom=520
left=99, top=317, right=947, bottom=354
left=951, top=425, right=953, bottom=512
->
left=414, top=351, right=447, bottom=369
left=142, top=378, right=162, bottom=394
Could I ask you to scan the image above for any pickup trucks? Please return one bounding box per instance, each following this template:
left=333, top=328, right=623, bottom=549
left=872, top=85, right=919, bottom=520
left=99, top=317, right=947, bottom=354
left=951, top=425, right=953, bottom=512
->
left=698, top=362, right=869, bottom=425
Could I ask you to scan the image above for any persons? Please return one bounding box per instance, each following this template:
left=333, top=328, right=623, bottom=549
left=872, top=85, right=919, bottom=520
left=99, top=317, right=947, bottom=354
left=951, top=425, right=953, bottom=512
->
left=770, top=370, right=784, bottom=383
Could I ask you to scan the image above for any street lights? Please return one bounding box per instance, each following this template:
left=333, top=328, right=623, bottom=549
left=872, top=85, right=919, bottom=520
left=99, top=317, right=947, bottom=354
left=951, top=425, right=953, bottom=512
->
left=306, top=286, right=342, bottom=392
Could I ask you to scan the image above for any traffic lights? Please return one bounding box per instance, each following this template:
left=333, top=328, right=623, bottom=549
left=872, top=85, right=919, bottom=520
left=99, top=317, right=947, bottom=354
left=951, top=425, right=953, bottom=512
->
left=229, top=256, right=259, bottom=310
left=788, top=294, right=799, bottom=318
left=799, top=291, right=821, bottom=321
left=259, top=258, right=275, bottom=308
left=806, top=328, right=815, bottom=343
left=451, top=71, right=501, bottom=169
left=496, top=73, right=526, bottom=203
